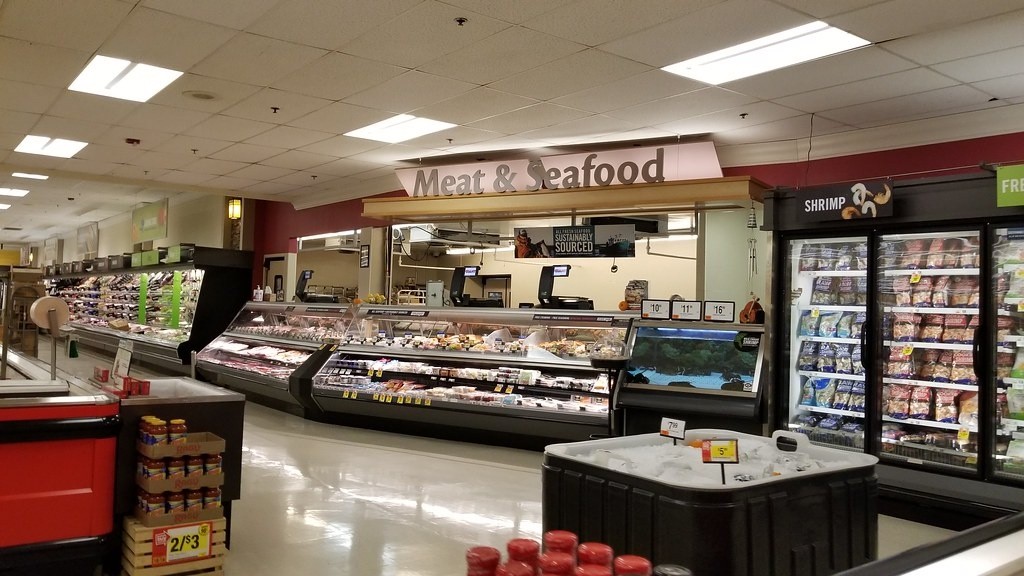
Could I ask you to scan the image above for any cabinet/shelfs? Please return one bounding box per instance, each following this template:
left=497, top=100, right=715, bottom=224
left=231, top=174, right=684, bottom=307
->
left=122, top=516, right=226, bottom=576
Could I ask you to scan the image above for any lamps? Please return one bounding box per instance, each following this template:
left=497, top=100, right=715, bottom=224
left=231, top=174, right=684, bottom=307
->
left=228, top=197, right=242, bottom=220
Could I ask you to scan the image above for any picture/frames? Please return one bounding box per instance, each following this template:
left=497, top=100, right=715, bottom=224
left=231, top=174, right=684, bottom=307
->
left=360, top=245, right=369, bottom=268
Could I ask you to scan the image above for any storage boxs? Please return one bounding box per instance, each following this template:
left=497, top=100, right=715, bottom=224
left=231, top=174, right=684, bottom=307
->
left=425, top=280, right=444, bottom=308
left=137, top=431, right=225, bottom=460
left=135, top=472, right=224, bottom=494
left=43, top=239, right=196, bottom=278
left=134, top=505, right=224, bottom=528
